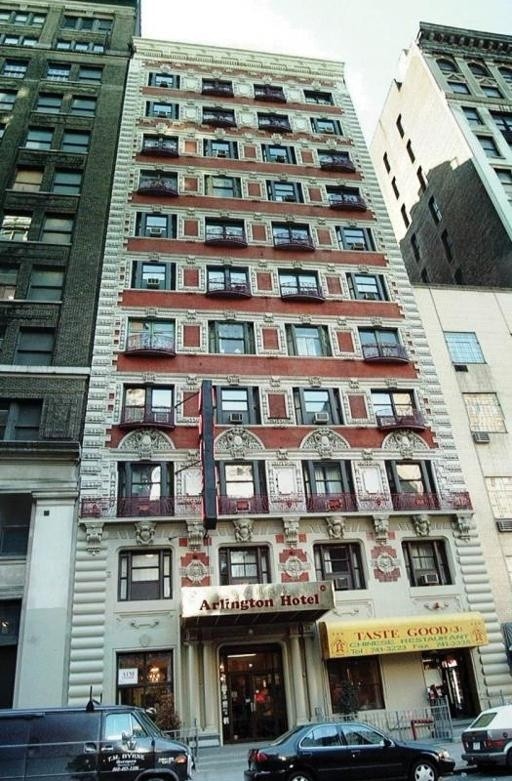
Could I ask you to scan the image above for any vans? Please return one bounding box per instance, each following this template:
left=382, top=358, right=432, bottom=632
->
left=0, top=688, right=195, bottom=781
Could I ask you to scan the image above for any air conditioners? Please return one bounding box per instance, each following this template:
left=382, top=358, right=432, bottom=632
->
left=150, top=228, right=162, bottom=237
left=229, top=412, right=244, bottom=423
left=147, top=277, right=160, bottom=289
left=334, top=577, right=350, bottom=590
left=352, top=241, right=365, bottom=251
left=313, top=412, right=330, bottom=424
left=158, top=110, right=167, bottom=118
left=217, top=149, right=226, bottom=157
left=420, top=573, right=439, bottom=585
left=365, top=292, right=376, bottom=300
left=325, top=126, right=334, bottom=133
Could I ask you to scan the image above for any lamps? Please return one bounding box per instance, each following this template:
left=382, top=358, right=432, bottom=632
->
left=146, top=663, right=161, bottom=683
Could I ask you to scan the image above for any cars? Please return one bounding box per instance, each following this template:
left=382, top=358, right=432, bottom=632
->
left=244, top=715, right=457, bottom=780
left=459, top=704, right=512, bottom=769
